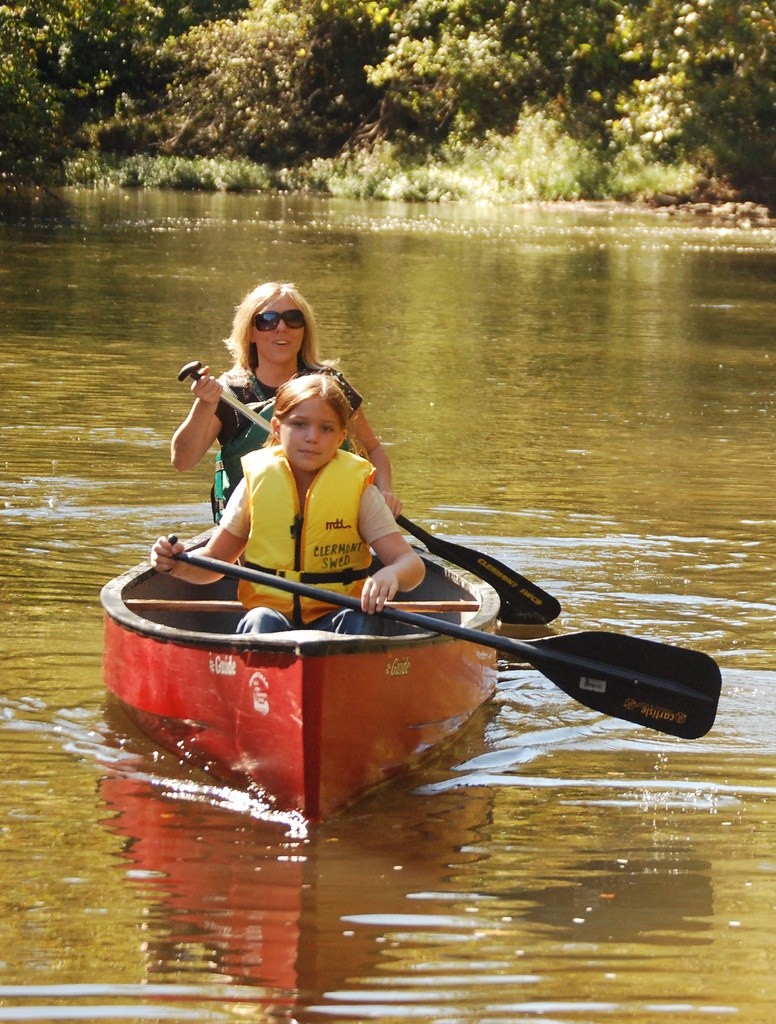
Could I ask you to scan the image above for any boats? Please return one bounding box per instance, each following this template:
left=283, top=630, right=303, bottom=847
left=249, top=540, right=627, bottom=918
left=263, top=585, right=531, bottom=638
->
left=97, top=518, right=503, bottom=826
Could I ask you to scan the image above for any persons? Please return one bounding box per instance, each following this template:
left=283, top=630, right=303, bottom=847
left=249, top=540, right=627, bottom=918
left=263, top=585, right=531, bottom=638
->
left=169, top=281, right=404, bottom=567
left=149, top=374, right=425, bottom=636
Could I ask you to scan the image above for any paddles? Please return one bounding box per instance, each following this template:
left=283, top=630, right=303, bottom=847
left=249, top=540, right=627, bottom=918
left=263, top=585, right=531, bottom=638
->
left=180, top=358, right=562, bottom=627
left=172, top=455, right=724, bottom=738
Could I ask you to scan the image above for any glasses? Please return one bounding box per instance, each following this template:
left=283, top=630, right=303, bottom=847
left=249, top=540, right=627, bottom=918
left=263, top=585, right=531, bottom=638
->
left=252, top=309, right=305, bottom=331
